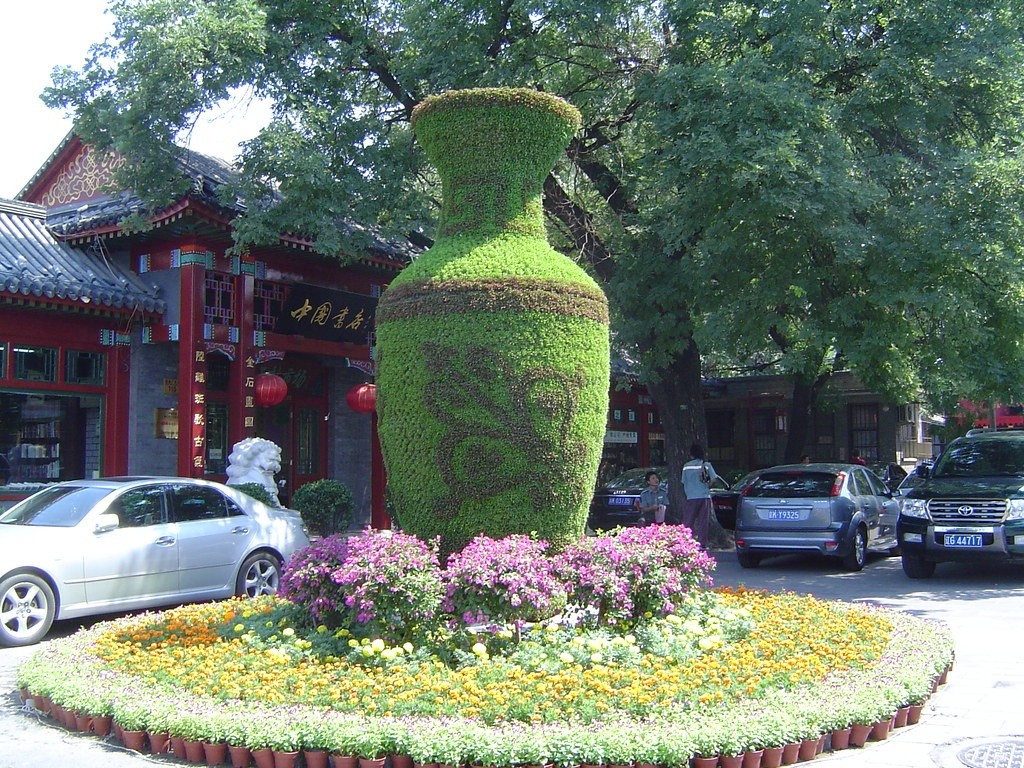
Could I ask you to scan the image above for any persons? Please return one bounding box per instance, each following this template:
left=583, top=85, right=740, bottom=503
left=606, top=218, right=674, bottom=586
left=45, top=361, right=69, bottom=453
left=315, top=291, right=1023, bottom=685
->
left=681, top=444, right=717, bottom=550
left=801, top=455, right=810, bottom=464
left=849, top=448, right=866, bottom=465
left=0, top=400, right=60, bottom=491
left=637, top=471, right=670, bottom=526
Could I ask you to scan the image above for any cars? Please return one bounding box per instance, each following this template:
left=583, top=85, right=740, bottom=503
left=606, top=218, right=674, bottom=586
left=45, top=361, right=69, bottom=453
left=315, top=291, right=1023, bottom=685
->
left=713, top=458, right=900, bottom=571
left=589, top=465, right=730, bottom=537
left=867, top=460, right=934, bottom=509
left=0, top=477, right=312, bottom=647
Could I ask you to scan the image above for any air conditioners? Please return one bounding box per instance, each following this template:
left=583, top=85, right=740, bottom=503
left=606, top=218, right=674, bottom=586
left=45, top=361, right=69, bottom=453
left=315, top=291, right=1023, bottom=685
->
left=898, top=404, right=912, bottom=423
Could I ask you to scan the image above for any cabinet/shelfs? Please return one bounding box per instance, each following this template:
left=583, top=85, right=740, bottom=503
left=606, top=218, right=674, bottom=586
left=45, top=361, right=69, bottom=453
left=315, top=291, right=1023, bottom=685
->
left=15, top=407, right=86, bottom=483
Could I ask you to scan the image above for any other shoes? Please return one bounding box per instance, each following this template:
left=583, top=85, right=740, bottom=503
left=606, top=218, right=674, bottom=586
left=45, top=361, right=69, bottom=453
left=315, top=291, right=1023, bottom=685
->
left=700, top=547, right=709, bottom=550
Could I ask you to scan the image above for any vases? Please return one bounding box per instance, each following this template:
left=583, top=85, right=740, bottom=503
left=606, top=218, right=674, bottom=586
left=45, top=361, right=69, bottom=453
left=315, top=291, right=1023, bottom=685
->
left=374, top=86, right=610, bottom=582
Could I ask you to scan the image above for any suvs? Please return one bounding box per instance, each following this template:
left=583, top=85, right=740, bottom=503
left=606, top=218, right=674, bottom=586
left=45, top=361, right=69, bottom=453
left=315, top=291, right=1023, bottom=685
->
left=897, top=427, right=1024, bottom=578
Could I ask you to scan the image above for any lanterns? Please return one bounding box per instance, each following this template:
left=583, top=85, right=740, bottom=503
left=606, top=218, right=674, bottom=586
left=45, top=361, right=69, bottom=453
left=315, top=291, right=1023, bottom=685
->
left=346, top=381, right=378, bottom=414
left=255, top=370, right=287, bottom=408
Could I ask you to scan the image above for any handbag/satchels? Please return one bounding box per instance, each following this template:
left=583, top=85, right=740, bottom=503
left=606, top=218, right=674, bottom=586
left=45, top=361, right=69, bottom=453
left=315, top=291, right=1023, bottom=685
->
left=701, top=461, right=710, bottom=483
